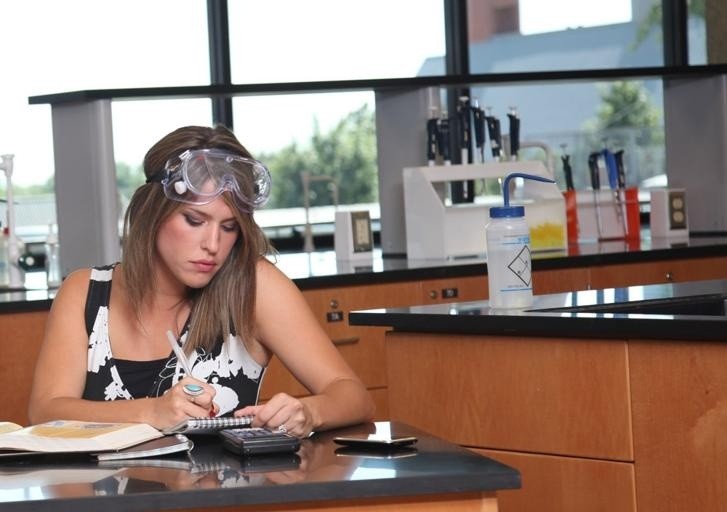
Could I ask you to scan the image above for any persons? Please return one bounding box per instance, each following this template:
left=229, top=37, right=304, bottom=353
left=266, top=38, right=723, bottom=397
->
left=27, top=124, right=377, bottom=440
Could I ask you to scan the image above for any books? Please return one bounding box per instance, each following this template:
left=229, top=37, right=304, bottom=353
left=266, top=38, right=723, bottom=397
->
left=0, top=408, right=257, bottom=461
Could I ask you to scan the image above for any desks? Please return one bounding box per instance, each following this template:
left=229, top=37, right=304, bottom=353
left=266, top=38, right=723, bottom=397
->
left=0, top=421, right=520, bottom=512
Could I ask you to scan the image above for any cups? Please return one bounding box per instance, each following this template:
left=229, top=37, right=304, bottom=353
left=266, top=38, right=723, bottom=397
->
left=484, top=172, right=556, bottom=309
left=332, top=210, right=354, bottom=261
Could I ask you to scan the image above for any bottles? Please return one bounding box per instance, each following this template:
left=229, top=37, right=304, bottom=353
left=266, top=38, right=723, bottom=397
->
left=0, top=228, right=26, bottom=288
left=43, top=235, right=63, bottom=289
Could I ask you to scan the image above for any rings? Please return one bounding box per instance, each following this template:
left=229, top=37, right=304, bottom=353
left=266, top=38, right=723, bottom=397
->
left=183, top=384, right=205, bottom=400
left=279, top=425, right=287, bottom=433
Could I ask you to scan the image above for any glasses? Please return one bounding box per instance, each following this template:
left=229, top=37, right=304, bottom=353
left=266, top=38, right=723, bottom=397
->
left=145, top=147, right=274, bottom=214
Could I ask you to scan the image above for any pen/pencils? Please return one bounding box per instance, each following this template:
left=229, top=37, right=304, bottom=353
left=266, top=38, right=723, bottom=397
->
left=165, top=329, right=215, bottom=420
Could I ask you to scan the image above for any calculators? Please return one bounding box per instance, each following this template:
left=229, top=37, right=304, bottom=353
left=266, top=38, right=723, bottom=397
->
left=216, top=426, right=302, bottom=456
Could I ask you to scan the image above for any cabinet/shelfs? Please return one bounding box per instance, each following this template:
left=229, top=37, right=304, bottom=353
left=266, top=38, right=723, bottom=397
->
left=420, top=266, right=590, bottom=304
left=589, top=257, right=727, bottom=288
left=258, top=281, right=421, bottom=443
